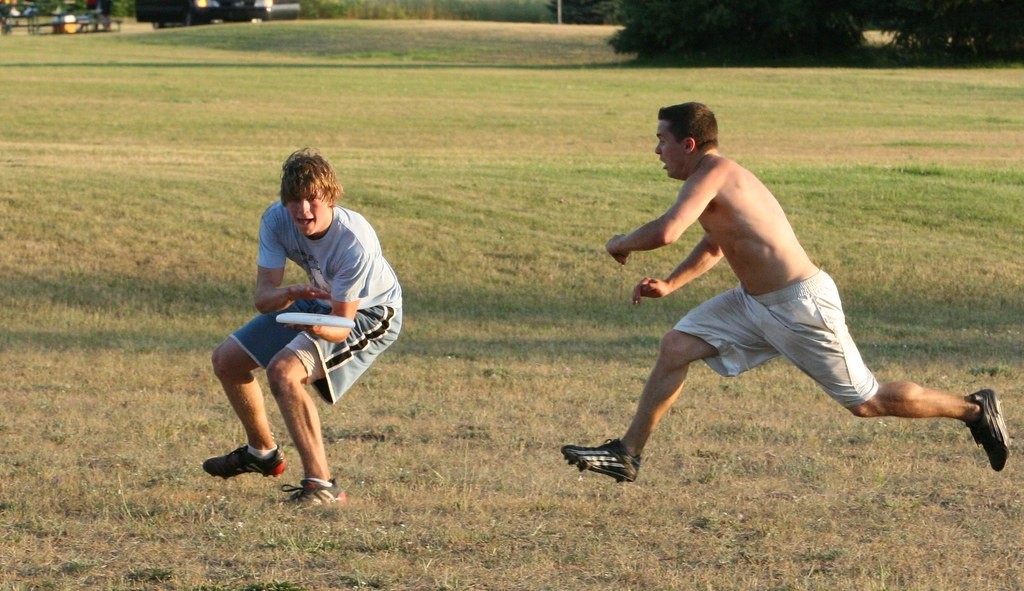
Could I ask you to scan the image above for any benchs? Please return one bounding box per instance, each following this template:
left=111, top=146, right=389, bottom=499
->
left=75, top=15, right=123, bottom=34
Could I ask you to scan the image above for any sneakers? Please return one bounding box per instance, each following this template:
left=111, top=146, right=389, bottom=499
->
left=965, top=389, right=1011, bottom=471
left=202, top=445, right=287, bottom=479
left=560, top=438, right=640, bottom=482
left=261, top=478, right=346, bottom=511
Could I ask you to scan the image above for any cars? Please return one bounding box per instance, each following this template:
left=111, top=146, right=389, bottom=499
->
left=134, top=0, right=301, bottom=30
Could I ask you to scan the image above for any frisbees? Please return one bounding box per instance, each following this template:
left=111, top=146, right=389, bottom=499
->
left=274, top=312, right=358, bottom=330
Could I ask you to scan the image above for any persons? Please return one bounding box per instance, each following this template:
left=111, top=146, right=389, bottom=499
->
left=560, top=101, right=1011, bottom=483
left=77, top=0, right=110, bottom=33
left=202, top=147, right=403, bottom=513
